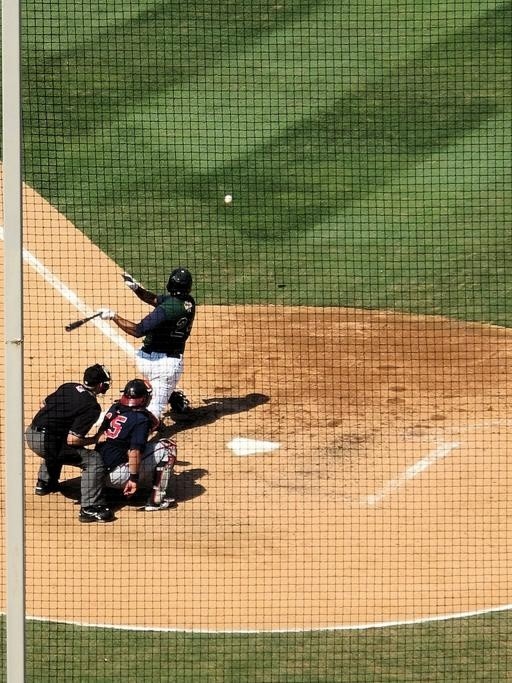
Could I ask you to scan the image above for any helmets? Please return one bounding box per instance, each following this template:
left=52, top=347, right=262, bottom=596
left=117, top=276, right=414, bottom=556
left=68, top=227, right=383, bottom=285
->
left=83, top=363, right=111, bottom=395
left=166, top=267, right=193, bottom=294
left=122, top=379, right=152, bottom=412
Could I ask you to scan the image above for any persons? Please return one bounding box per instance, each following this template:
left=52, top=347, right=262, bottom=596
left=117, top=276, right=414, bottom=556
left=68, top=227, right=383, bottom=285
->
left=97, top=378, right=179, bottom=511
left=101, top=267, right=194, bottom=436
left=26, top=364, right=116, bottom=524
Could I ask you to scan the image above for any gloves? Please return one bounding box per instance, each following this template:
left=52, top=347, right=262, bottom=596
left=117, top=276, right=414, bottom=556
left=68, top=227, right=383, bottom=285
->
left=96, top=307, right=116, bottom=321
left=123, top=273, right=139, bottom=292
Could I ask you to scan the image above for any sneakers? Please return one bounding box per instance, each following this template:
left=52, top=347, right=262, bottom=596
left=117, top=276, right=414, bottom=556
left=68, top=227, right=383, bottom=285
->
left=78, top=503, right=116, bottom=522
left=172, top=390, right=186, bottom=416
left=145, top=499, right=170, bottom=513
left=35, top=478, right=59, bottom=498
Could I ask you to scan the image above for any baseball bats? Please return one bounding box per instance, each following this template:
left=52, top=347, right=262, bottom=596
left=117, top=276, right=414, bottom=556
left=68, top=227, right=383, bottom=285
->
left=66, top=313, right=102, bottom=332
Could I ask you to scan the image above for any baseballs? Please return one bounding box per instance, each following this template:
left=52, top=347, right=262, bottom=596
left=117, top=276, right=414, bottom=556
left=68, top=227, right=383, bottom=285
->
left=225, top=195, right=232, bottom=203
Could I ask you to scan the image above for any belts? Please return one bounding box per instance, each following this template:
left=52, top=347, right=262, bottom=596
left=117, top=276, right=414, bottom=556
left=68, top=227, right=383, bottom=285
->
left=29, top=423, right=63, bottom=437
left=141, top=346, right=182, bottom=359
